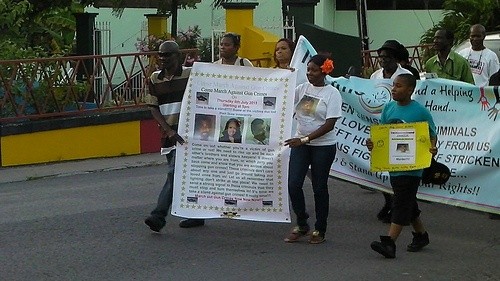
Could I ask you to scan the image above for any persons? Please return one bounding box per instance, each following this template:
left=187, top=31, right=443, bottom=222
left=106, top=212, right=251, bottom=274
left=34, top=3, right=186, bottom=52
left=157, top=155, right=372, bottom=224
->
left=267, top=37, right=297, bottom=70
left=143, top=41, right=205, bottom=231
left=285, top=53, right=343, bottom=244
left=211, top=32, right=254, bottom=67
left=363, top=23, right=500, bottom=224
left=194, top=114, right=270, bottom=145
left=369, top=73, right=438, bottom=258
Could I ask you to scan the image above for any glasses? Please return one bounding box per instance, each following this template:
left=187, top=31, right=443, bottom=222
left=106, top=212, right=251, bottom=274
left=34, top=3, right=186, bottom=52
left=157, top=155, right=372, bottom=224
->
left=157, top=51, right=176, bottom=58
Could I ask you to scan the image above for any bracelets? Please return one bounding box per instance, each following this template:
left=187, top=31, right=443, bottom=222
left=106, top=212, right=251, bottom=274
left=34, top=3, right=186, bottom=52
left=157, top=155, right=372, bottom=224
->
left=300, top=135, right=310, bottom=145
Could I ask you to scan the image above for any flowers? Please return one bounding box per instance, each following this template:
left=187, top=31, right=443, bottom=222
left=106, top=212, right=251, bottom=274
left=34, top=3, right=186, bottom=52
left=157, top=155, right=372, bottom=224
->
left=135, top=25, right=202, bottom=63
left=320, top=57, right=334, bottom=74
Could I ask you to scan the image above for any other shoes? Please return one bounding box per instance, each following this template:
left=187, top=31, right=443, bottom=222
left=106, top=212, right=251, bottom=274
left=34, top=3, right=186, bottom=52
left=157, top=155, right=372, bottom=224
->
left=179, top=218, right=204, bottom=228
left=489, top=213, right=499, bottom=220
left=377, top=206, right=392, bottom=224
left=307, top=230, right=326, bottom=244
left=406, top=230, right=430, bottom=252
left=370, top=235, right=397, bottom=258
left=145, top=215, right=166, bottom=232
left=284, top=225, right=309, bottom=242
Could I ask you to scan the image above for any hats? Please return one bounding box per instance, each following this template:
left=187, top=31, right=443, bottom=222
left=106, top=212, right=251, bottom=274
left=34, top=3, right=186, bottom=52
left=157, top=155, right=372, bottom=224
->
left=377, top=40, right=403, bottom=61
left=400, top=44, right=411, bottom=63
